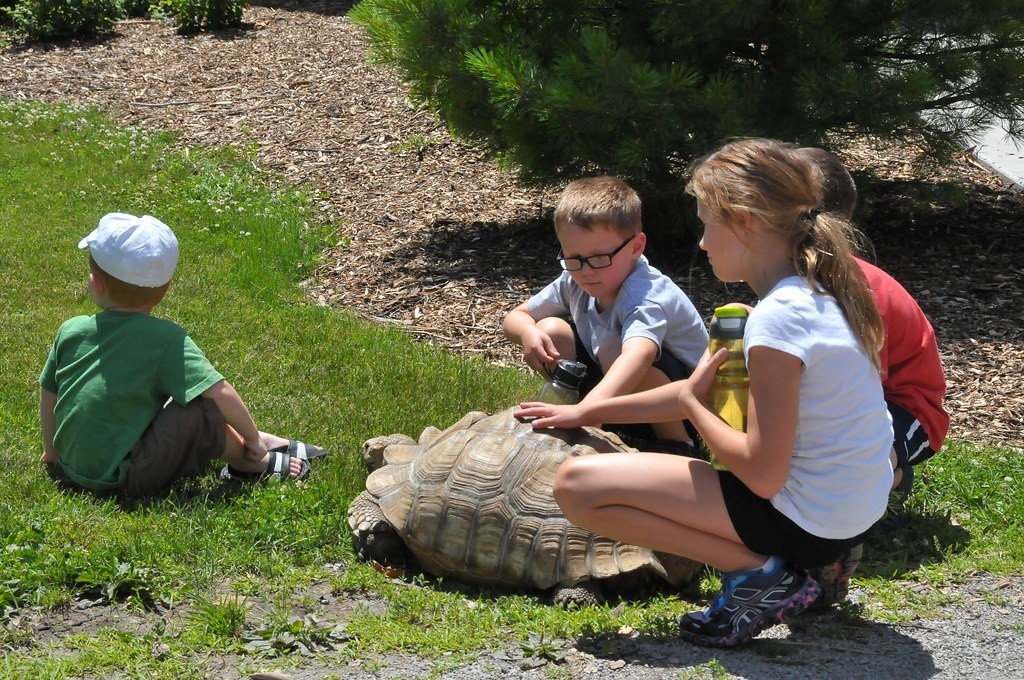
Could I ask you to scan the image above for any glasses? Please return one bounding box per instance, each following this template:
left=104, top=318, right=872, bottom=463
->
left=556, top=234, right=637, bottom=271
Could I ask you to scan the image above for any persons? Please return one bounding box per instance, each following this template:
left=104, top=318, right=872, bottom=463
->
left=502, top=176, right=710, bottom=458
left=37, top=213, right=326, bottom=509
left=511, top=138, right=950, bottom=647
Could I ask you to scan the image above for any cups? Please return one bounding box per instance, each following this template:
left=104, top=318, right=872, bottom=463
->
left=710, top=303, right=754, bottom=470
left=538, top=359, right=587, bottom=406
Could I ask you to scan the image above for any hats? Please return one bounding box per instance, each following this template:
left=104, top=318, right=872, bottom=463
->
left=77, top=213, right=180, bottom=288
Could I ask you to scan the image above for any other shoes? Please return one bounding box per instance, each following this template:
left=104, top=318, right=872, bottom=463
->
left=892, top=464, right=914, bottom=504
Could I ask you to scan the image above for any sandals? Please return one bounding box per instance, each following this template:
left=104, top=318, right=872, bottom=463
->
left=220, top=451, right=311, bottom=487
left=268, top=438, right=327, bottom=460
left=652, top=434, right=702, bottom=458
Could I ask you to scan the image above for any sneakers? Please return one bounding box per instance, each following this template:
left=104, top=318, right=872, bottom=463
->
left=813, top=542, right=864, bottom=609
left=680, top=553, right=822, bottom=648
